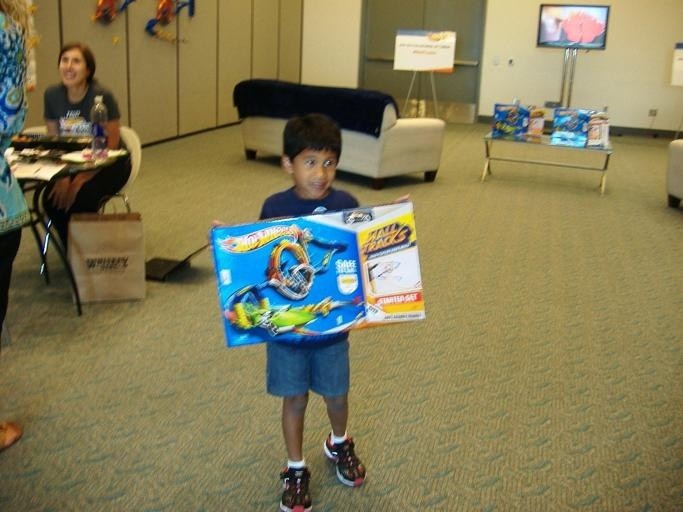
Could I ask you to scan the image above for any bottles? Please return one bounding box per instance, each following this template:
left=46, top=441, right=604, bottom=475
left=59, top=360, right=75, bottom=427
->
left=89, top=93, right=109, bottom=157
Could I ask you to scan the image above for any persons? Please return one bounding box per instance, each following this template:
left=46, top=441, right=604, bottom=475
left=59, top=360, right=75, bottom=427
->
left=1, top=0, right=33, bottom=344
left=208, top=112, right=412, bottom=511
left=41, top=43, right=134, bottom=263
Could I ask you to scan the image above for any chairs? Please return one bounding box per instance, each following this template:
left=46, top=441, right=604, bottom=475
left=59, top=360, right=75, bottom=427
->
left=36, top=125, right=141, bottom=276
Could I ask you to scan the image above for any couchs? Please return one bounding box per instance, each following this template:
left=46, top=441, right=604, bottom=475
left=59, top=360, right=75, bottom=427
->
left=666, top=138, right=682, bottom=206
left=232, top=77, right=446, bottom=189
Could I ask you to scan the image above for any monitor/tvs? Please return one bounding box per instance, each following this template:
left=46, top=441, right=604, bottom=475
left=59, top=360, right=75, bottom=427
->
left=537, top=4, right=611, bottom=51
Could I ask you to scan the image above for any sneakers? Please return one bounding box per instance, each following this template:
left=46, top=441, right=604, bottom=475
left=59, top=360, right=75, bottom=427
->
left=279, top=467, right=313, bottom=512
left=1, top=420, right=22, bottom=451
left=323, top=432, right=366, bottom=487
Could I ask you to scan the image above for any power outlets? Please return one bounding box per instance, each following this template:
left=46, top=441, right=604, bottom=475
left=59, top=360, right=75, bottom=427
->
left=648, top=109, right=657, bottom=116
